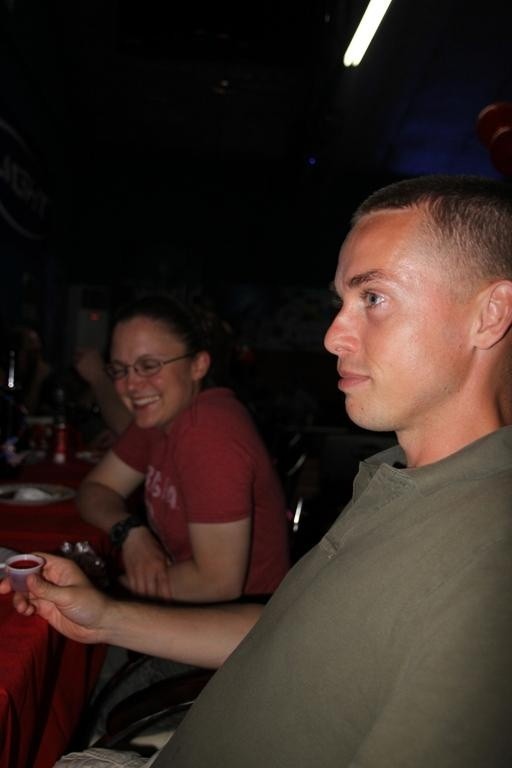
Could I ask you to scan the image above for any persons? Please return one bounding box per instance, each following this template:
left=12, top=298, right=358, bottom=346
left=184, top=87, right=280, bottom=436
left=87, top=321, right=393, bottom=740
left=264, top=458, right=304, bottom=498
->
left=15, top=317, right=55, bottom=400
left=0, top=97, right=510, bottom=767
left=74, top=345, right=134, bottom=450
left=73, top=296, right=294, bottom=753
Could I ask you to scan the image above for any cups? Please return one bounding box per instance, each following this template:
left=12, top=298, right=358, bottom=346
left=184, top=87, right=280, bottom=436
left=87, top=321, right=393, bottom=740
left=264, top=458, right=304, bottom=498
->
left=6, top=555, right=46, bottom=592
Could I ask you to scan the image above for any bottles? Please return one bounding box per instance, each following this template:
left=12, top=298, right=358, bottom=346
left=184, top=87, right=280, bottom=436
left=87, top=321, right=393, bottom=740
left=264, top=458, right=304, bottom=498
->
left=55, top=422, right=68, bottom=466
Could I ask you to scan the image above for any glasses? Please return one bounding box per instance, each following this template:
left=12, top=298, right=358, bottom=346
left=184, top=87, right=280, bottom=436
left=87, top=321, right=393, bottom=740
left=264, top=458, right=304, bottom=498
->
left=105, top=353, right=194, bottom=380
